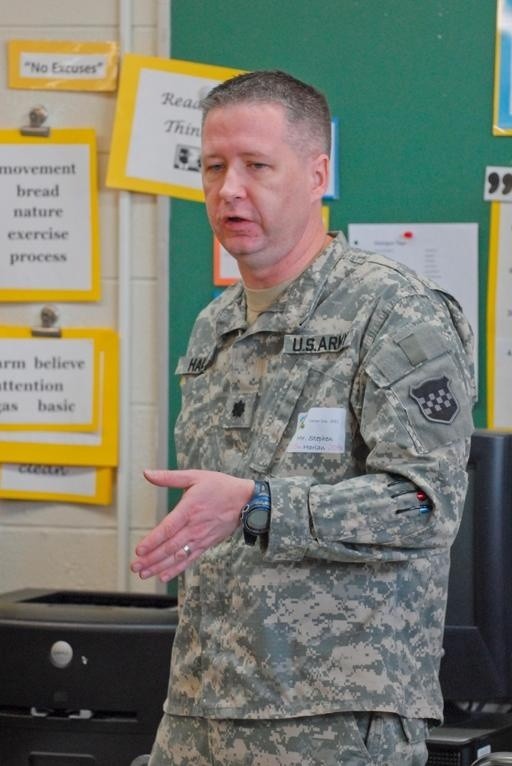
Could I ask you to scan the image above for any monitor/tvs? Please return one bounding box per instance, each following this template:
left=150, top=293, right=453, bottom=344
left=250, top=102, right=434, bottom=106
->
left=438, top=431, right=512, bottom=702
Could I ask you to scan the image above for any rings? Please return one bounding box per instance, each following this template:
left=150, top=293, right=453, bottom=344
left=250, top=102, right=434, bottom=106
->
left=182, top=544, right=191, bottom=554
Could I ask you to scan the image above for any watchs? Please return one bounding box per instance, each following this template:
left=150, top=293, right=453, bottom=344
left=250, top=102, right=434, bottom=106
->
left=240, top=479, right=270, bottom=546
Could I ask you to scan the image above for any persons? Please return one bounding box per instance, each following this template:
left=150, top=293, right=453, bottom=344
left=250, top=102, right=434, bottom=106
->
left=128, top=67, right=477, bottom=766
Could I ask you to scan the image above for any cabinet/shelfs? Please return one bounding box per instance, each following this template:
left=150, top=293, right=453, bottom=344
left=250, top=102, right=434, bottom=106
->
left=0, top=588, right=179, bottom=766
